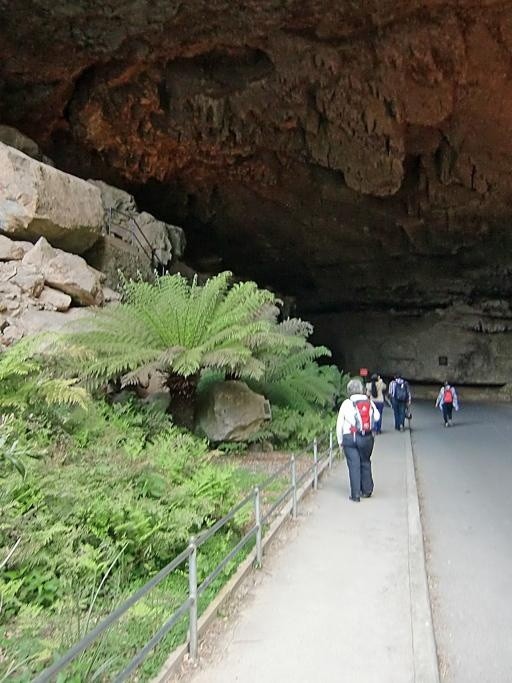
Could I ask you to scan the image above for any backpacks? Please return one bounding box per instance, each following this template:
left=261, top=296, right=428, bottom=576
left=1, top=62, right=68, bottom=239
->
left=348, top=396, right=374, bottom=436
left=442, top=386, right=453, bottom=404
left=394, top=380, right=409, bottom=402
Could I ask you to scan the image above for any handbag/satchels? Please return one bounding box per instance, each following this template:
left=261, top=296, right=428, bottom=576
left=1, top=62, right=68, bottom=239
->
left=405, top=406, right=412, bottom=419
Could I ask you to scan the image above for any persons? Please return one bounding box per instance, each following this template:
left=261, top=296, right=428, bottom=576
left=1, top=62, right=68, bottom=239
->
left=364, top=372, right=389, bottom=435
left=336, top=377, right=382, bottom=500
left=434, top=378, right=459, bottom=427
left=386, top=372, right=411, bottom=431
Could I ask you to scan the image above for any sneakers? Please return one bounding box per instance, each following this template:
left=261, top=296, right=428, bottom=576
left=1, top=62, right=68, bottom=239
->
left=348, top=494, right=360, bottom=502
left=395, top=423, right=404, bottom=432
left=362, top=490, right=372, bottom=497
left=375, top=430, right=382, bottom=435
left=445, top=419, right=454, bottom=427
